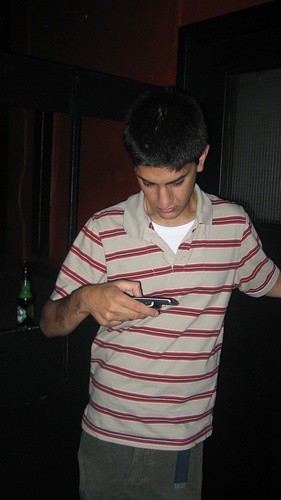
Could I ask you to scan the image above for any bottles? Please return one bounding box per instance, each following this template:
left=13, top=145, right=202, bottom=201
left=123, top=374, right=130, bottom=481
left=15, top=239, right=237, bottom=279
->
left=17, top=267, right=35, bottom=328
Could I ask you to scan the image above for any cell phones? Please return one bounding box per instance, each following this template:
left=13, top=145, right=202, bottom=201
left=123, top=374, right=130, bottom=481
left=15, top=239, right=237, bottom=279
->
left=133, top=297, right=179, bottom=309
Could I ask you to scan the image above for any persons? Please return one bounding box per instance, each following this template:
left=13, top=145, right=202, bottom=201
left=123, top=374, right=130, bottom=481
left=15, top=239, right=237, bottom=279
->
left=40, top=89, right=281, bottom=500
